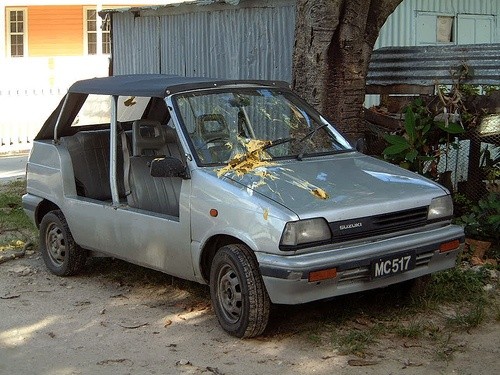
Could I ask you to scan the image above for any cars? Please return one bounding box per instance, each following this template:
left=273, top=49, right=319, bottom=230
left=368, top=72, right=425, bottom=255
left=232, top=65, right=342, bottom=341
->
left=20, top=73, right=466, bottom=340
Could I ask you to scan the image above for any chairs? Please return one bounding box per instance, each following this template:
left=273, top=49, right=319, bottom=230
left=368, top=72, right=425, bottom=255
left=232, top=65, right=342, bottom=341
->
left=119, top=117, right=185, bottom=217
left=64, top=128, right=127, bottom=201
left=197, top=111, right=233, bottom=163
